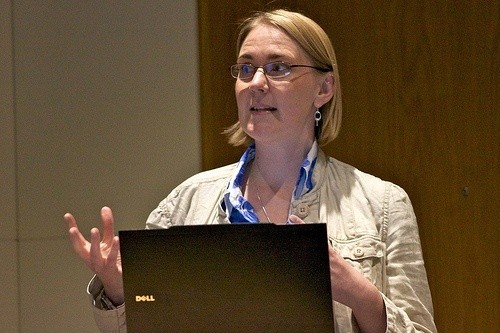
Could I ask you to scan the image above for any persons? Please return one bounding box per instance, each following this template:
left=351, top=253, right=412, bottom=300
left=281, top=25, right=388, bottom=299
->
left=64, top=9, right=437, bottom=333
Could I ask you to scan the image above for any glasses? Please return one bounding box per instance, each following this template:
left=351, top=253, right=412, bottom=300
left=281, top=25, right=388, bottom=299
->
left=228, top=61, right=322, bottom=79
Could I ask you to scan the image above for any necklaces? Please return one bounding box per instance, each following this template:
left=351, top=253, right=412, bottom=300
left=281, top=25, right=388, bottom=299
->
left=249, top=162, right=274, bottom=224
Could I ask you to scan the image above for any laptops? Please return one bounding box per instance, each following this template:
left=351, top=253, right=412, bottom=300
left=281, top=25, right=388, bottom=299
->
left=119, top=222, right=336, bottom=333
